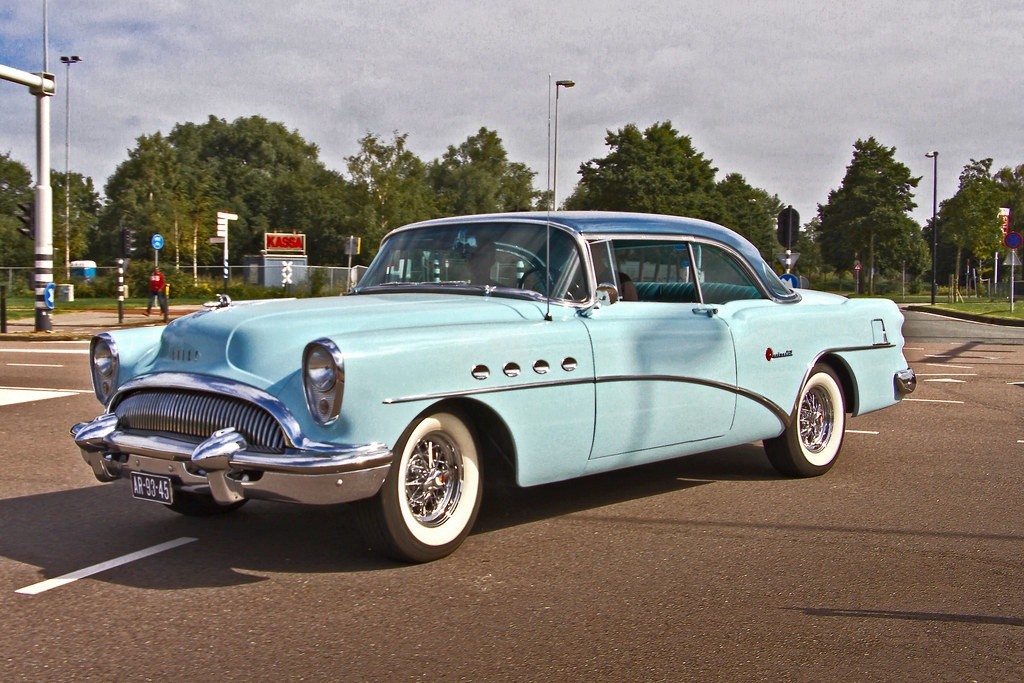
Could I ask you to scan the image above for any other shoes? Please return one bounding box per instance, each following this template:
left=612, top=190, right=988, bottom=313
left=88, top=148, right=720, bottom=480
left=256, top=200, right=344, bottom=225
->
left=160, top=312, right=164, bottom=316
left=142, top=312, right=149, bottom=316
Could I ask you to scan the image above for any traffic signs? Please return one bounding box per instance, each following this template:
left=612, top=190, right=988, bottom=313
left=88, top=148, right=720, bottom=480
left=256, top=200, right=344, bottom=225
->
left=209, top=211, right=239, bottom=243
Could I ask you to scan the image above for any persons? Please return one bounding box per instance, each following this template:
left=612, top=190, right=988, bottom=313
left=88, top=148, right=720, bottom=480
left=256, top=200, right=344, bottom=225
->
left=142, top=267, right=166, bottom=316
left=589, top=241, right=639, bottom=301
left=464, top=238, right=507, bottom=286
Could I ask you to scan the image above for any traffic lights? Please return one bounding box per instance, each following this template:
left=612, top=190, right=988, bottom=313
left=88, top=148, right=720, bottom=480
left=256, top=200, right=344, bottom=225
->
left=15, top=200, right=35, bottom=240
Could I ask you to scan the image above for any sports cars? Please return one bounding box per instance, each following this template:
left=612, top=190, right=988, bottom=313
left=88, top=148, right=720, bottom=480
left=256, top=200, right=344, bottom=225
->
left=69, top=210, right=921, bottom=563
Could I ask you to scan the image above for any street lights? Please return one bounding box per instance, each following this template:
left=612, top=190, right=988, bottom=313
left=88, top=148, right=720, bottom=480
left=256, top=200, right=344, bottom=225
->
left=552, top=78, right=576, bottom=212
left=59, top=54, right=83, bottom=282
left=924, top=150, right=939, bottom=304
left=747, top=197, right=757, bottom=241
